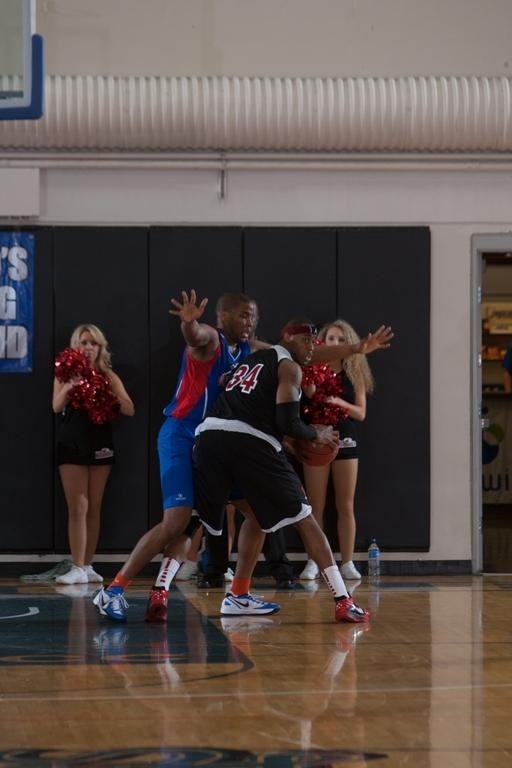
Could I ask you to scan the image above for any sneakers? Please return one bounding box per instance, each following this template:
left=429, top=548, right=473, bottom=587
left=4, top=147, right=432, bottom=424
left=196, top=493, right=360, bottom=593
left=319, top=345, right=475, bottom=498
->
left=148, top=586, right=168, bottom=609
left=93, top=585, right=129, bottom=621
left=55, top=567, right=104, bottom=584
left=171, top=561, right=234, bottom=588
left=341, top=561, right=361, bottom=579
left=334, top=595, right=370, bottom=623
left=276, top=579, right=305, bottom=589
left=300, top=559, right=320, bottom=579
left=220, top=591, right=280, bottom=615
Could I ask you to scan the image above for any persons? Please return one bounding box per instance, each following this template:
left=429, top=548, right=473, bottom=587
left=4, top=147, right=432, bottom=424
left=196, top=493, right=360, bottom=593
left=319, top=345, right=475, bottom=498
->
left=175, top=499, right=235, bottom=582
left=197, top=508, right=306, bottom=590
left=51, top=323, right=136, bottom=584
left=299, top=319, right=375, bottom=580
left=54, top=585, right=97, bottom=677
left=501, top=342, right=512, bottom=393
left=144, top=314, right=371, bottom=624
left=91, top=288, right=394, bottom=623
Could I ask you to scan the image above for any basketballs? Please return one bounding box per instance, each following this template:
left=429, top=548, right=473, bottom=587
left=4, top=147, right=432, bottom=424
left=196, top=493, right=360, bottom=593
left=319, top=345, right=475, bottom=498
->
left=294, top=423, right=340, bottom=467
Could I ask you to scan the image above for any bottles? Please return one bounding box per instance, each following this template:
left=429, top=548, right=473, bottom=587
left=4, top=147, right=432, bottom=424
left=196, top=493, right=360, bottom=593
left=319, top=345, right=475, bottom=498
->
left=368, top=585, right=380, bottom=623
left=367, top=538, right=380, bottom=585
